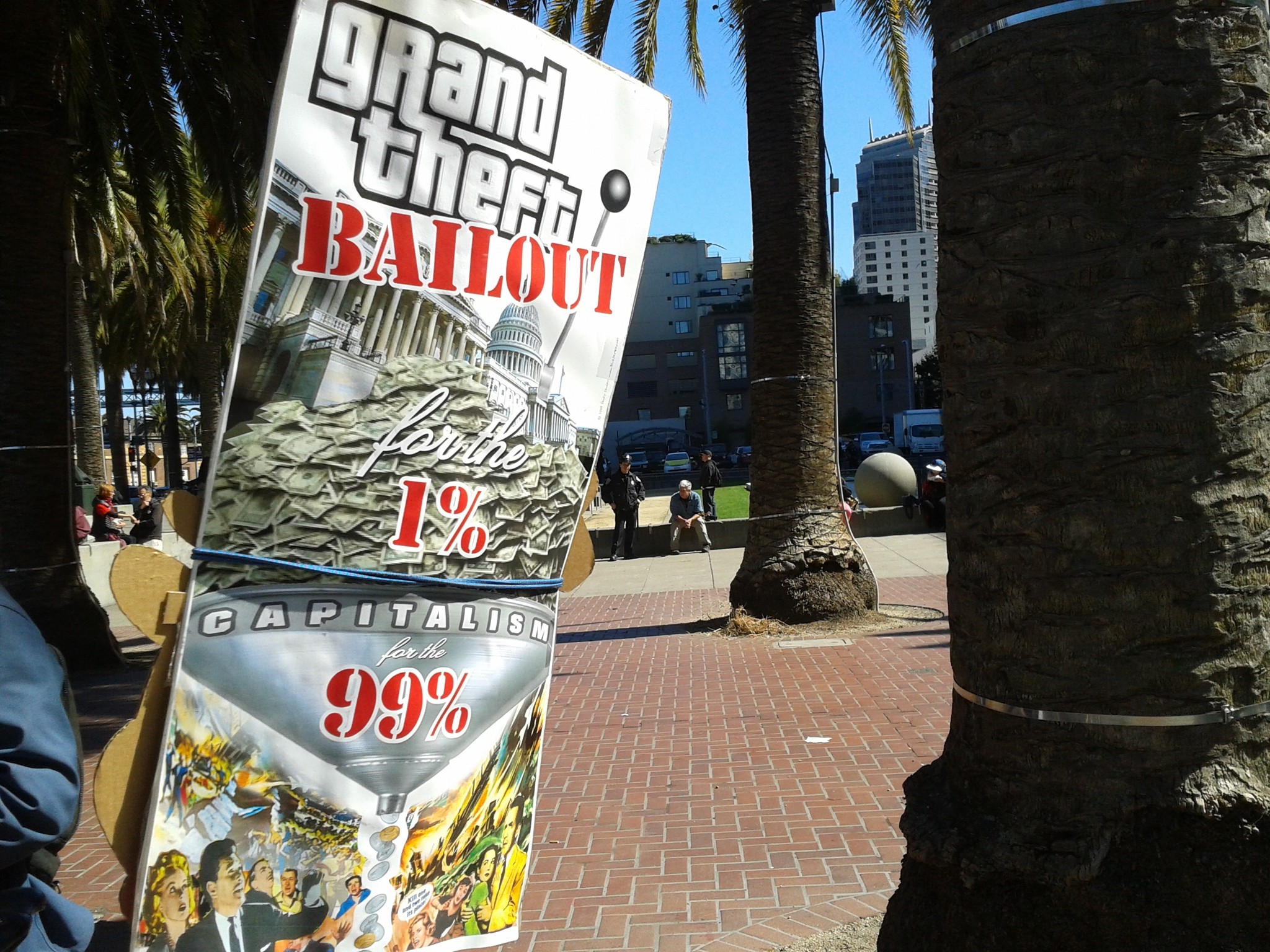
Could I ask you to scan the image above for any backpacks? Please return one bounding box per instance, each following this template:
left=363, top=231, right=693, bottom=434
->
left=710, top=461, right=722, bottom=487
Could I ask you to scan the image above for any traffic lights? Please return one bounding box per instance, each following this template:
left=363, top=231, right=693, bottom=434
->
left=128, top=447, right=135, bottom=462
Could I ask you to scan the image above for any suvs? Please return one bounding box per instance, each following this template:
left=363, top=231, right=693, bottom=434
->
left=699, top=444, right=733, bottom=468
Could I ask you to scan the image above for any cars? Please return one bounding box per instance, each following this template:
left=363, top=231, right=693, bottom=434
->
left=648, top=451, right=666, bottom=470
left=627, top=452, right=649, bottom=472
left=663, top=452, right=694, bottom=472
left=854, top=431, right=893, bottom=460
left=730, top=447, right=744, bottom=463
left=736, top=446, right=752, bottom=466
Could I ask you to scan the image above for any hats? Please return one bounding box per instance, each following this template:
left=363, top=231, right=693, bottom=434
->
left=701, top=450, right=712, bottom=457
left=926, top=459, right=945, bottom=472
left=620, top=455, right=631, bottom=464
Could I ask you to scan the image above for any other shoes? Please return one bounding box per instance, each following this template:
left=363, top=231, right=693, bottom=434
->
left=610, top=554, right=617, bottom=560
left=672, top=550, right=680, bottom=555
left=624, top=555, right=637, bottom=559
left=702, top=544, right=710, bottom=552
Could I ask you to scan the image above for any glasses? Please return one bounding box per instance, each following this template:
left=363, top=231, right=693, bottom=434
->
left=138, top=492, right=148, bottom=498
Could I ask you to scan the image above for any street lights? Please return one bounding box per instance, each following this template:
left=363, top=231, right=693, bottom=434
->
left=130, top=399, right=139, bottom=442
left=126, top=416, right=133, bottom=444
left=191, top=417, right=197, bottom=444
left=123, top=390, right=160, bottom=485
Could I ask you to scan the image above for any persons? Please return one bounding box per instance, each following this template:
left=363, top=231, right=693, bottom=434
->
left=0, top=584, right=96, bottom=952
left=836, top=476, right=852, bottom=525
left=838, top=443, right=845, bottom=469
left=699, top=450, right=717, bottom=521
left=75, top=503, right=91, bottom=543
left=601, top=454, right=644, bottom=560
left=129, top=485, right=163, bottom=551
left=922, top=460, right=947, bottom=513
left=91, top=484, right=138, bottom=545
left=669, top=480, right=712, bottom=554
left=845, top=441, right=858, bottom=473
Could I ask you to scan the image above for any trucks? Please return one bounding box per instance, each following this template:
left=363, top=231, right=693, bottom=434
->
left=893, top=408, right=945, bottom=457
